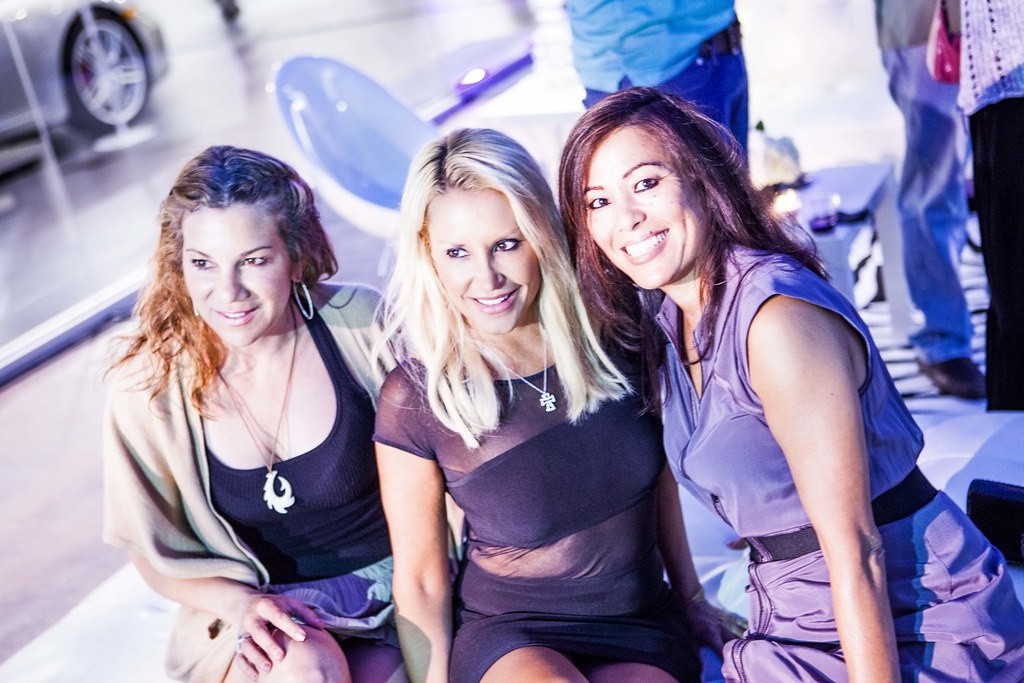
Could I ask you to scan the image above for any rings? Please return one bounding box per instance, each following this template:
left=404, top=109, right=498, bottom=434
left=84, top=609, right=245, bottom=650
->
left=236, top=635, right=251, bottom=655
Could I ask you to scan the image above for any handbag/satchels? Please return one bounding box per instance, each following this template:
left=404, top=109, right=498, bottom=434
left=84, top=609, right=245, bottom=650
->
left=926, top=0, right=960, bottom=85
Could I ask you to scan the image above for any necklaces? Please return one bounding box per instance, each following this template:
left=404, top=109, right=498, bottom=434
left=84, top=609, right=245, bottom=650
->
left=217, top=299, right=297, bottom=514
left=479, top=331, right=556, bottom=413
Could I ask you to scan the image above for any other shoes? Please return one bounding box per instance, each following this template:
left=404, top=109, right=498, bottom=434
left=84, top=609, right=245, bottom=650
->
left=916, top=357, right=985, bottom=402
left=222, top=5, right=238, bottom=22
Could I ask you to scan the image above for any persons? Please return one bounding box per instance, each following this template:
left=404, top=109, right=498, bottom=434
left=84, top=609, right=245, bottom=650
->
left=370, top=128, right=750, bottom=683
left=103, top=145, right=465, bottom=683
left=875, top=1, right=1024, bottom=418
left=565, top=1, right=749, bottom=154
left=557, top=88, right=1024, bottom=683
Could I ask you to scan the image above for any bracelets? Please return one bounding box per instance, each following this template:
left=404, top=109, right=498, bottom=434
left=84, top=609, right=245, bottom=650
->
left=685, top=586, right=704, bottom=607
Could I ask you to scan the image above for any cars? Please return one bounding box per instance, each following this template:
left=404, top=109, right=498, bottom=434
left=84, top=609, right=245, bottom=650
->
left=0, top=1, right=170, bottom=152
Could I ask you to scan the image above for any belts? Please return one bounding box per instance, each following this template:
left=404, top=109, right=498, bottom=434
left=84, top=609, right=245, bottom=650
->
left=724, top=465, right=939, bottom=563
